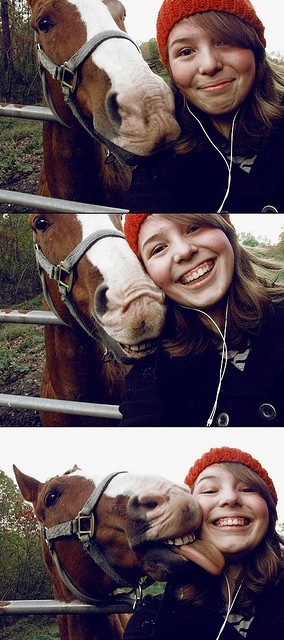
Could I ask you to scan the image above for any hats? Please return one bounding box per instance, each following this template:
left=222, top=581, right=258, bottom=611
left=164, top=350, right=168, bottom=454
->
left=184, top=445, right=278, bottom=507
left=156, top=0, right=267, bottom=73
left=124, top=214, right=234, bottom=259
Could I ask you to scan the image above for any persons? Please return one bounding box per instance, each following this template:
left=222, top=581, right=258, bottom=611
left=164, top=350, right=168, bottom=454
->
left=127, top=1, right=284, bottom=213
left=123, top=447, right=284, bottom=638
left=115, top=214, right=283, bottom=426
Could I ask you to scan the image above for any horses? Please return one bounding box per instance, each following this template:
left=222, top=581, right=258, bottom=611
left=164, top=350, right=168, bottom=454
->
left=25, top=0, right=183, bottom=210
left=28, top=210, right=168, bottom=426
left=9, top=462, right=228, bottom=638
